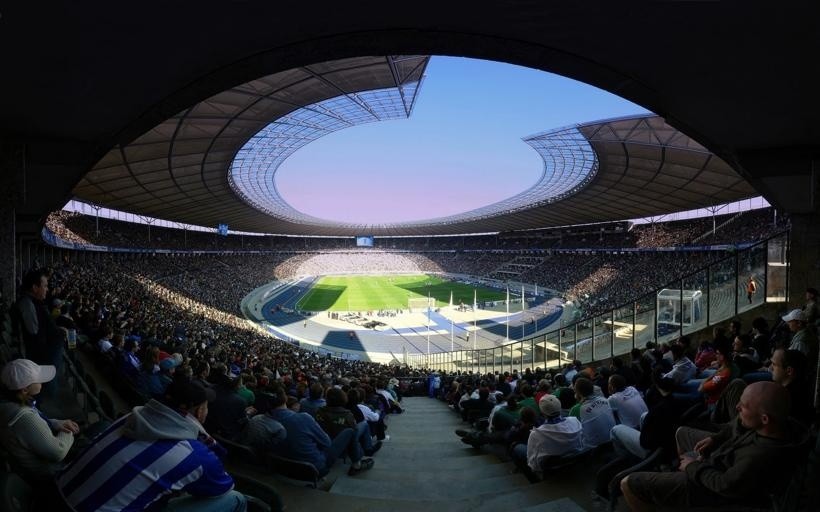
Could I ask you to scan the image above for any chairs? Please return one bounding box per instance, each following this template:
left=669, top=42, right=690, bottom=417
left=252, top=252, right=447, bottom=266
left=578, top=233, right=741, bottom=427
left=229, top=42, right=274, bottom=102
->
left=0, top=212, right=820, bottom=512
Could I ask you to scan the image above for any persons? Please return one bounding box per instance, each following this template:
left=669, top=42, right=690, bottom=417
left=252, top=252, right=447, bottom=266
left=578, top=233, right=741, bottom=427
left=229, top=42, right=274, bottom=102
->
left=0, top=208, right=820, bottom=512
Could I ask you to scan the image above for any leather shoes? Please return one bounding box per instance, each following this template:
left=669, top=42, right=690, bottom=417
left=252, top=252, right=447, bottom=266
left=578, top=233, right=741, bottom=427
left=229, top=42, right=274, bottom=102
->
left=365, top=442, right=382, bottom=456
left=456, top=430, right=472, bottom=440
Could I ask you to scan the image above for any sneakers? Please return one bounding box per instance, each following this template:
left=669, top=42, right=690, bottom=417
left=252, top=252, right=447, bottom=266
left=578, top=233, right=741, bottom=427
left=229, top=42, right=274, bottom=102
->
left=347, top=458, right=374, bottom=477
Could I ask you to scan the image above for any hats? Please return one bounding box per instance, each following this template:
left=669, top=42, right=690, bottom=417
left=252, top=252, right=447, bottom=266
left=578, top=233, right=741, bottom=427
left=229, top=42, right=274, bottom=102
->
left=0, top=358, right=57, bottom=392
left=165, top=379, right=217, bottom=411
left=781, top=308, right=808, bottom=322
left=535, top=390, right=561, bottom=417
